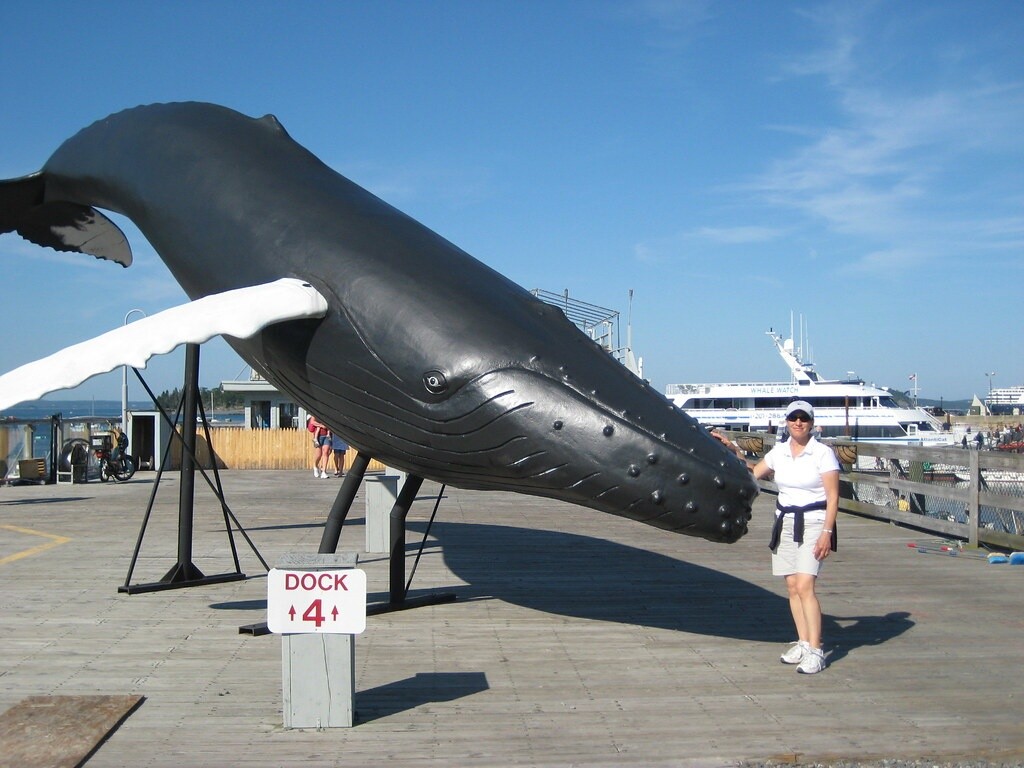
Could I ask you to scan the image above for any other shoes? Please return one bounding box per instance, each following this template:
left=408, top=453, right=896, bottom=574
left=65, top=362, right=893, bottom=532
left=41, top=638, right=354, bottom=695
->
left=312, top=439, right=320, bottom=446
left=334, top=471, right=342, bottom=477
left=326, top=434, right=332, bottom=441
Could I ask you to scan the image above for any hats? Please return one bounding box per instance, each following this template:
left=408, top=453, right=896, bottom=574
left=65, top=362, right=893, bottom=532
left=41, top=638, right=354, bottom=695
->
left=784, top=400, right=815, bottom=420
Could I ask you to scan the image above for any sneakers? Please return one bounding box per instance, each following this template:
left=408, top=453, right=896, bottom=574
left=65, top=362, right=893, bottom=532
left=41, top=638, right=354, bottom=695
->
left=320, top=472, right=329, bottom=478
left=313, top=467, right=320, bottom=477
left=796, top=646, right=827, bottom=674
left=779, top=639, right=810, bottom=665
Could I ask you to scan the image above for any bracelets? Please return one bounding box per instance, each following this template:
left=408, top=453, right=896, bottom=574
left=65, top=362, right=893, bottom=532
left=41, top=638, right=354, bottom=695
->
left=822, top=529, right=833, bottom=533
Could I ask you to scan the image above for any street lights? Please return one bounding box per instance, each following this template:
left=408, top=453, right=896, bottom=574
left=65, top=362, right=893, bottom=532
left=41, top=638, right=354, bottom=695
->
left=985, top=372, right=995, bottom=413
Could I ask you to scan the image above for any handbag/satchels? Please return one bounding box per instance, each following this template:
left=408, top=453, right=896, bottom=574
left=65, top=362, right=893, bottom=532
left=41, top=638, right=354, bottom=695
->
left=308, top=417, right=315, bottom=433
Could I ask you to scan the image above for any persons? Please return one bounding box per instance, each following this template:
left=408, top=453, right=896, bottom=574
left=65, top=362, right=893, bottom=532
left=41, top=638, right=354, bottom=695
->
left=897, top=494, right=911, bottom=512
left=942, top=421, right=1024, bottom=451
left=308, top=416, right=350, bottom=480
left=710, top=400, right=841, bottom=674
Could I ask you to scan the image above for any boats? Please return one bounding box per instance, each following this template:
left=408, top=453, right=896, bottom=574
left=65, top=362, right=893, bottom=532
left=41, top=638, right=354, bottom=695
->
left=664, top=310, right=954, bottom=478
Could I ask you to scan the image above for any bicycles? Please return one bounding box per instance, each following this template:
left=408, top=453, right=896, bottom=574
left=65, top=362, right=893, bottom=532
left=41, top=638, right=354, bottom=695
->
left=94, top=430, right=136, bottom=482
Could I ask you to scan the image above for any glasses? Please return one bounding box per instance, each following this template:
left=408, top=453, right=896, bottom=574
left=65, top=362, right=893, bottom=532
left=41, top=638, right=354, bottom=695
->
left=788, top=414, right=810, bottom=423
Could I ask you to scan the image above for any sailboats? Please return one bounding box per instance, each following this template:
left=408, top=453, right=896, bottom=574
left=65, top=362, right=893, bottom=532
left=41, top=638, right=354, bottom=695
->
left=196, top=391, right=217, bottom=424
